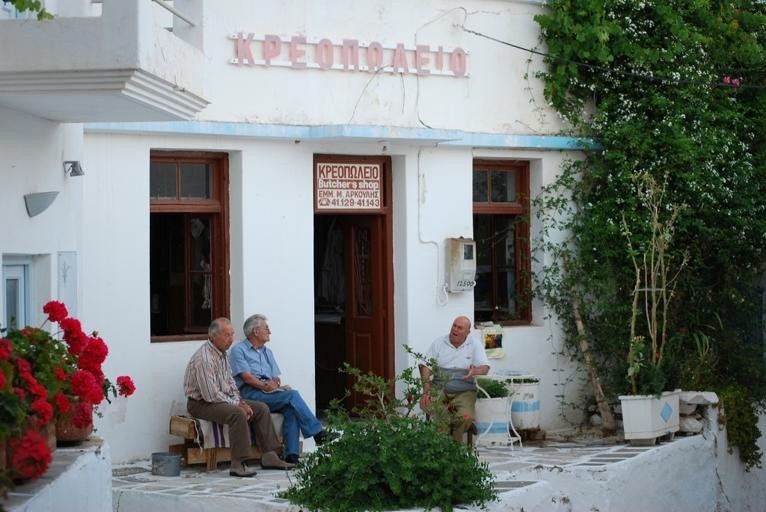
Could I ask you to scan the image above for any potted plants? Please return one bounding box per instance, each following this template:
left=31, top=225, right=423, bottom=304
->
left=616, top=336, right=681, bottom=444
left=472, top=376, right=514, bottom=444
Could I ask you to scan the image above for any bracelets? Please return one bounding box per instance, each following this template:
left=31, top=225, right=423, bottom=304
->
left=275, top=379, right=280, bottom=387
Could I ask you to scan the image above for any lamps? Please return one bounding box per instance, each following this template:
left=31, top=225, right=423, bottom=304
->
left=62, top=159, right=85, bottom=177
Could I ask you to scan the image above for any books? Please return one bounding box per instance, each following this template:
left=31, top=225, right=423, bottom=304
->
left=261, top=388, right=285, bottom=394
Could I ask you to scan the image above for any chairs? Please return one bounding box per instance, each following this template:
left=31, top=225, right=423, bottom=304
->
left=415, top=363, right=478, bottom=454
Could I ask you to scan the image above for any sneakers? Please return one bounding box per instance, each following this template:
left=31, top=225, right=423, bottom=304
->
left=314, top=431, right=340, bottom=446
left=228, top=458, right=258, bottom=477
left=261, top=450, right=296, bottom=471
left=286, top=453, right=305, bottom=468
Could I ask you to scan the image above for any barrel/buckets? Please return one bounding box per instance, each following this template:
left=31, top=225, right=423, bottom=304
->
left=474, top=396, right=511, bottom=443
left=147, top=452, right=182, bottom=477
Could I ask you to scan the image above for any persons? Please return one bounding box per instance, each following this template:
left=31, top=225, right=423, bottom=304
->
left=228, top=313, right=341, bottom=469
left=183, top=316, right=298, bottom=478
left=416, top=313, right=491, bottom=437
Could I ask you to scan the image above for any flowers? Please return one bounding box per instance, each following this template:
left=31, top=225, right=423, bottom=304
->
left=1, top=299, right=135, bottom=511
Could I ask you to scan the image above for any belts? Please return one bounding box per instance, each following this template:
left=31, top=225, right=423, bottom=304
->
left=237, top=376, right=271, bottom=392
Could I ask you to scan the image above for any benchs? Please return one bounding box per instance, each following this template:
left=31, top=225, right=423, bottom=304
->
left=167, top=410, right=303, bottom=474
